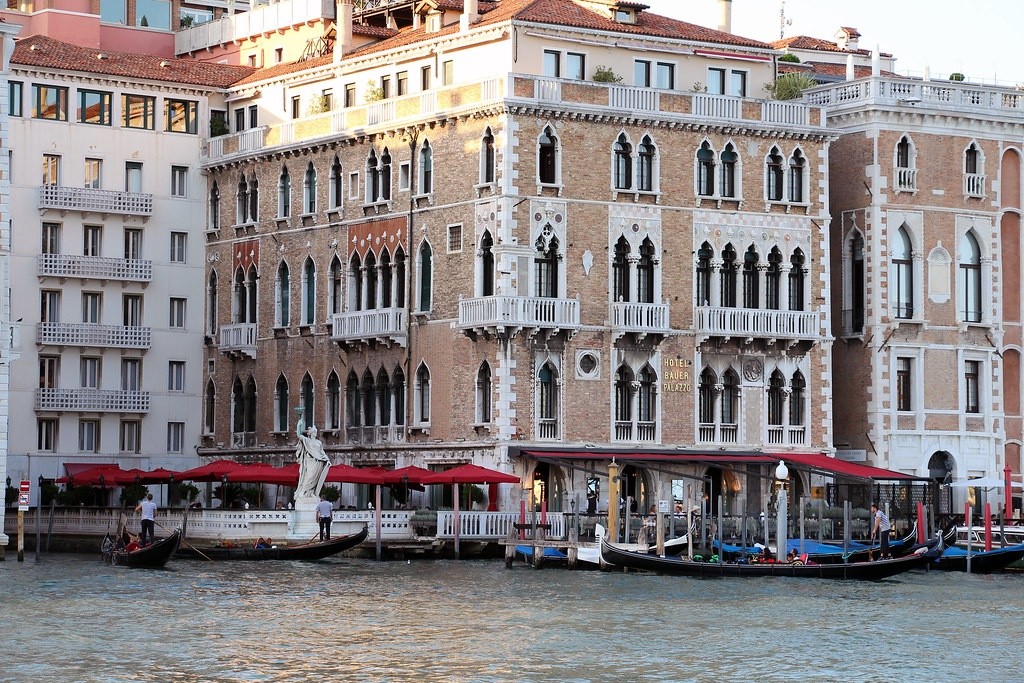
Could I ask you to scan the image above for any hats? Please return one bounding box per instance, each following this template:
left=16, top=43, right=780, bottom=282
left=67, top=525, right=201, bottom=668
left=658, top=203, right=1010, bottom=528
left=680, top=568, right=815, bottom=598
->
left=691, top=505, right=700, bottom=511
left=676, top=504, right=684, bottom=510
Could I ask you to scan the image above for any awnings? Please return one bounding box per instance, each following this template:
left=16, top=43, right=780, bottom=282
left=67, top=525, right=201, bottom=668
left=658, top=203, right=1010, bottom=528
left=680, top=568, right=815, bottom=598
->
left=64, top=463, right=120, bottom=488
left=772, top=453, right=935, bottom=553
left=526, top=451, right=792, bottom=481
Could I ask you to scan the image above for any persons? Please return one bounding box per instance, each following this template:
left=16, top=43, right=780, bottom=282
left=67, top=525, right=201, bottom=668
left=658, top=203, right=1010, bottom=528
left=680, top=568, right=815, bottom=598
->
left=673, top=506, right=684, bottom=518
left=990, top=504, right=1024, bottom=526
left=293, top=418, right=332, bottom=498
left=759, top=545, right=805, bottom=566
left=871, top=504, right=893, bottom=561
left=646, top=504, right=657, bottom=520
left=136, top=533, right=142, bottom=545
left=315, top=495, right=333, bottom=544
left=135, top=494, right=157, bottom=549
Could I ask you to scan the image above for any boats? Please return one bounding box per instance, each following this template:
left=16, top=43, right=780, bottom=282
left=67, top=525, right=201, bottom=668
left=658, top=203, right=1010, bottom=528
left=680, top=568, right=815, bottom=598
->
left=101, top=525, right=181, bottom=569
left=600, top=532, right=944, bottom=579
left=950, top=525, right=1024, bottom=569
left=712, top=521, right=958, bottom=563
left=176, top=523, right=369, bottom=560
left=576, top=535, right=688, bottom=570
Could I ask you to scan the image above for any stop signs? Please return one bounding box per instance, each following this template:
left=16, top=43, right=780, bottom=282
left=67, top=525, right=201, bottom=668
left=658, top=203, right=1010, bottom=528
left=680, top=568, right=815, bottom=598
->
left=19, top=480, right=30, bottom=492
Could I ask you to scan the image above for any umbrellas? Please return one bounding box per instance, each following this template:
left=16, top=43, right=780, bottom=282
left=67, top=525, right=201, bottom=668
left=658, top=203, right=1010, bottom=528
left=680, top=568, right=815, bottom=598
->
left=47, top=456, right=523, bottom=512
left=948, top=475, right=1024, bottom=525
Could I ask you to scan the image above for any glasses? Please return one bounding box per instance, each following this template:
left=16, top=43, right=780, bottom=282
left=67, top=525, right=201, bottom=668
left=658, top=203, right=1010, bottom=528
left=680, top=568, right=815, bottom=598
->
left=277, top=505, right=280, bottom=507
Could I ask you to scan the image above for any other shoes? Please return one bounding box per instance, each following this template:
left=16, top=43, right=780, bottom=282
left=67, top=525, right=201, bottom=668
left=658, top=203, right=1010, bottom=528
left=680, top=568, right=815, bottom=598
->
left=319, top=539, right=331, bottom=545
